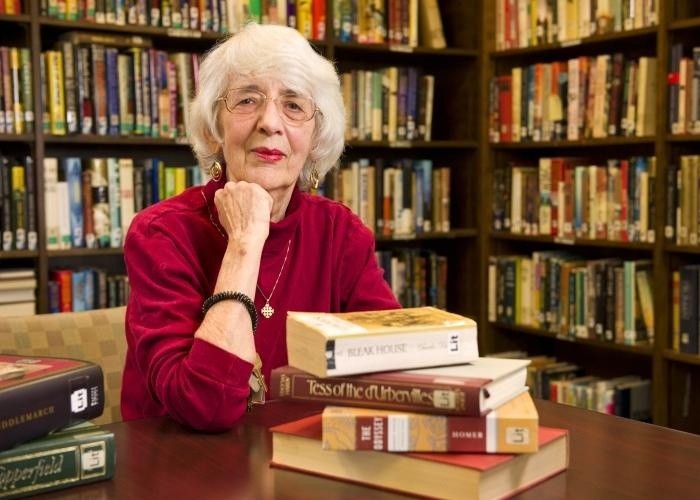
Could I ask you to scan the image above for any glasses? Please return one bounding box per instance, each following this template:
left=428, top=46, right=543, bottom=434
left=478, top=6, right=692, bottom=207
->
left=216, top=88, right=323, bottom=121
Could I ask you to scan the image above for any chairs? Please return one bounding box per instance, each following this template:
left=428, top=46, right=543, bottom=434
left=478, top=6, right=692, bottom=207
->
left=1, top=305, right=128, bottom=428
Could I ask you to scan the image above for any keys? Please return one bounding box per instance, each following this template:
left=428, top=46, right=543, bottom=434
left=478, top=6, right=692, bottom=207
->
left=247, top=395, right=252, bottom=413
left=252, top=352, right=268, bottom=393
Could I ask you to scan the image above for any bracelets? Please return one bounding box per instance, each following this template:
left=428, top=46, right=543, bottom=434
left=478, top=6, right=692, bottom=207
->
left=201, top=290, right=258, bottom=334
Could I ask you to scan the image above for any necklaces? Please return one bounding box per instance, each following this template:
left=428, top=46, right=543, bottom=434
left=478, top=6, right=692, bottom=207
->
left=200, top=188, right=291, bottom=319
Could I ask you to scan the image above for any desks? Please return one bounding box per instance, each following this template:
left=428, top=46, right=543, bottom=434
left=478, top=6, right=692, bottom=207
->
left=19, top=398, right=700, bottom=500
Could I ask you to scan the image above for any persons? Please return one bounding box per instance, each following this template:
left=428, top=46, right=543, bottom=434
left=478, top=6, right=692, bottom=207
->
left=120, top=20, right=404, bottom=433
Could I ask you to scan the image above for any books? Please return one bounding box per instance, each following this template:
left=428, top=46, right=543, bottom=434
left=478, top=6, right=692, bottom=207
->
left=0, top=353, right=105, bottom=454
left=39, top=0, right=327, bottom=41
left=337, top=158, right=451, bottom=237
left=0, top=0, right=22, bottom=16
left=40, top=41, right=203, bottom=143
left=0, top=46, right=34, bottom=136
left=376, top=248, right=447, bottom=312
left=488, top=53, right=657, bottom=144
left=334, top=1, right=419, bottom=48
left=338, top=66, right=434, bottom=142
left=0, top=422, right=116, bottom=500
left=271, top=356, right=531, bottom=418
left=495, top=0, right=660, bottom=53
left=419, top=0, right=447, bottom=50
left=485, top=350, right=653, bottom=421
left=10, top=164, right=25, bottom=250
left=492, top=155, right=658, bottom=244
left=43, top=155, right=203, bottom=252
left=47, top=267, right=130, bottom=313
left=286, top=306, right=479, bottom=379
left=322, top=391, right=539, bottom=454
left=0, top=267, right=39, bottom=316
left=267, top=412, right=570, bottom=500
left=671, top=264, right=700, bottom=354
left=667, top=43, right=700, bottom=134
left=664, top=155, right=700, bottom=247
left=487, top=249, right=656, bottom=347
left=0, top=158, right=10, bottom=250
left=25, top=157, right=39, bottom=252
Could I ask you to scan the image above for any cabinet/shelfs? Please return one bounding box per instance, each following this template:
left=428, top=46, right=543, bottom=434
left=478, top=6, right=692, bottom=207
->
left=0, top=0, right=476, bottom=321
left=474, top=0, right=699, bottom=436
